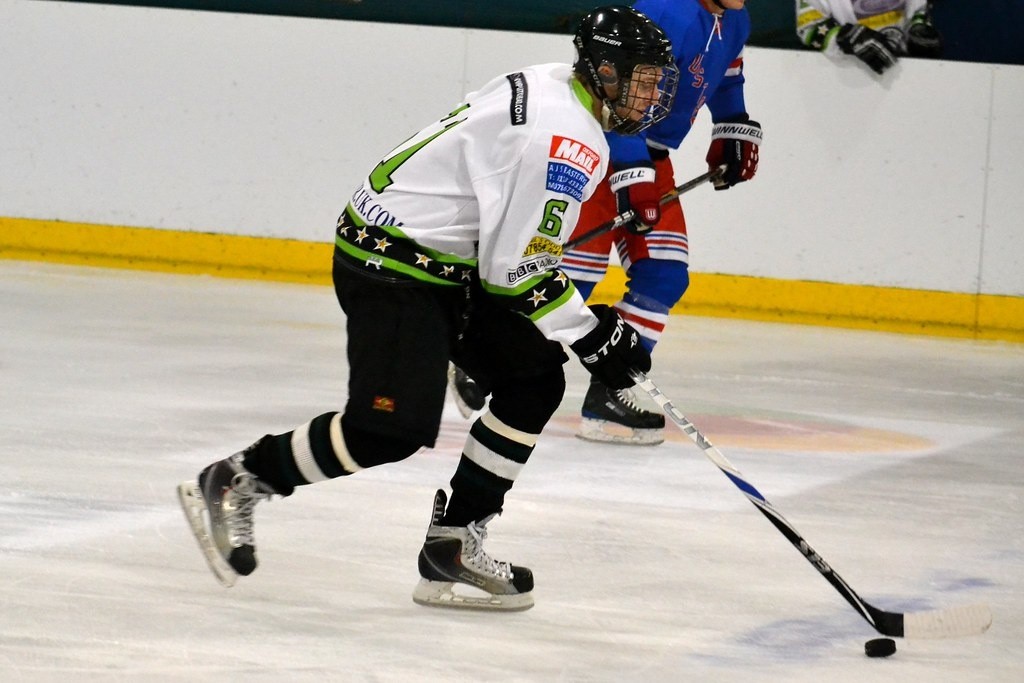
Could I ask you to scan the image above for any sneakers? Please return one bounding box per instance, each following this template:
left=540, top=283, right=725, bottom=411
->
left=412, top=490, right=535, bottom=612
left=447, top=362, right=486, bottom=419
left=178, top=433, right=275, bottom=589
left=575, top=374, right=666, bottom=446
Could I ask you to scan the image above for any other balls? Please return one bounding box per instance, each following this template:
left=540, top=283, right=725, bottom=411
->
left=865, top=639, right=898, bottom=657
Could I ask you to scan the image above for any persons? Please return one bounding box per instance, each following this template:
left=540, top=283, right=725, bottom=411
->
left=795, top=0, right=940, bottom=75
left=177, top=1, right=680, bottom=611
left=448, top=0, right=761, bottom=445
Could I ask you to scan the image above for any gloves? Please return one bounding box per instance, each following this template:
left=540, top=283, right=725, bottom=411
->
left=835, top=22, right=899, bottom=75
left=706, top=119, right=762, bottom=191
left=608, top=159, right=661, bottom=235
left=906, top=23, right=945, bottom=58
left=568, top=304, right=651, bottom=390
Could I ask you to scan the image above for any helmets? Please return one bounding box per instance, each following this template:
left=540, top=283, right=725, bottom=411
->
left=573, top=4, right=680, bottom=136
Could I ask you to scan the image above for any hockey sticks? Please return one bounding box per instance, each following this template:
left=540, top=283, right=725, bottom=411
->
left=561, top=162, right=731, bottom=255
left=627, top=366, right=993, bottom=637
